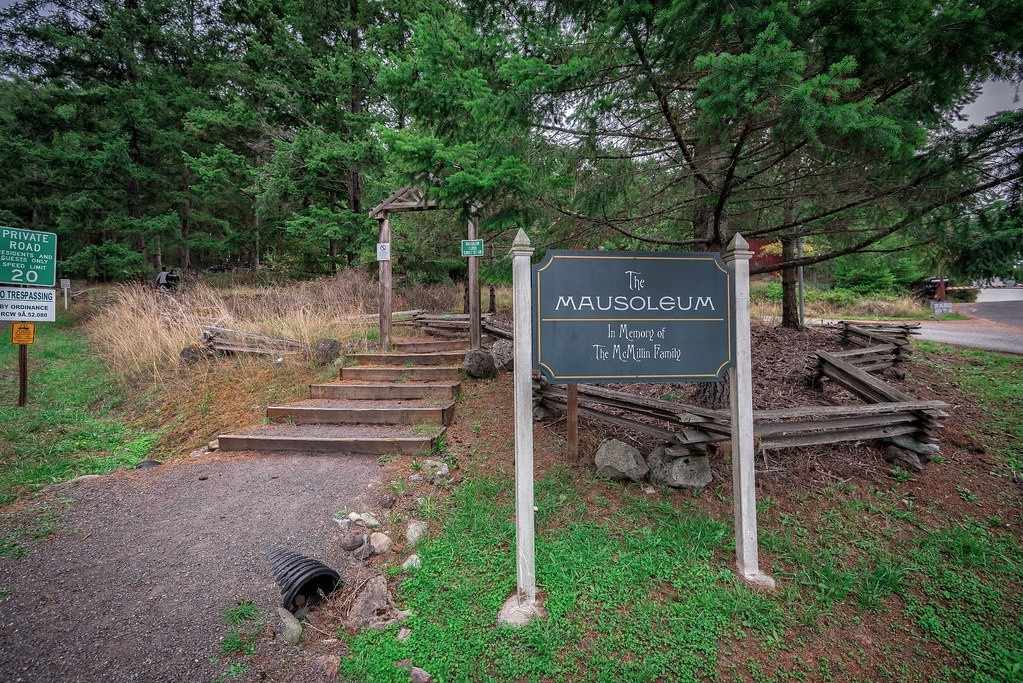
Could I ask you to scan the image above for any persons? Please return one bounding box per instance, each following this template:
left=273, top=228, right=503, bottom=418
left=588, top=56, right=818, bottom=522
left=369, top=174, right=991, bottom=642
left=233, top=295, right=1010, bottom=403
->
left=155, top=267, right=180, bottom=292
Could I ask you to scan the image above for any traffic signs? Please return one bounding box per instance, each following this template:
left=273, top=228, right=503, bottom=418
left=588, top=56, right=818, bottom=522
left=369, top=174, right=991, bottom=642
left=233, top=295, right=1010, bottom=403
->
left=0, top=226, right=58, bottom=287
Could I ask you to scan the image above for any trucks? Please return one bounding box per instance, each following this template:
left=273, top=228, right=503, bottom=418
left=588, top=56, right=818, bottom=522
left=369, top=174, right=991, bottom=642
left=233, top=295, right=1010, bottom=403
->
left=924, top=276, right=981, bottom=302
